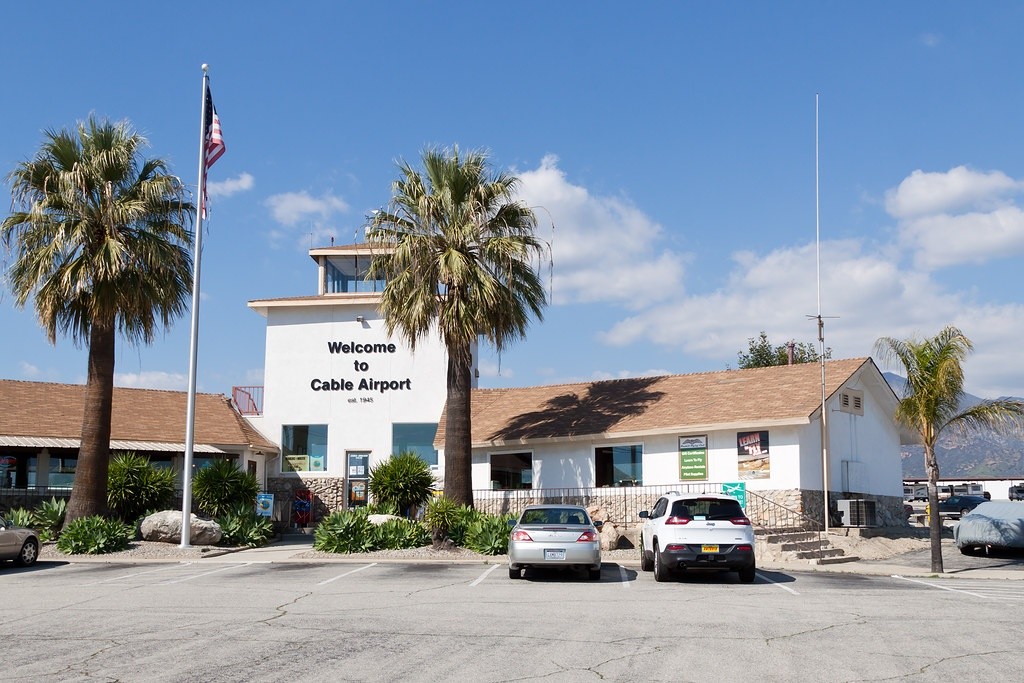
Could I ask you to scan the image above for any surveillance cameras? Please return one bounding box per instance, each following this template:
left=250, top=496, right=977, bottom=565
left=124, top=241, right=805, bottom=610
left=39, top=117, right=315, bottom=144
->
left=357, top=316, right=364, bottom=322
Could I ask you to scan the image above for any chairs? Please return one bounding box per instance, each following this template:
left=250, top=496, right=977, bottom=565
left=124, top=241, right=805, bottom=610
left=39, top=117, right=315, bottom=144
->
left=567, top=516, right=580, bottom=524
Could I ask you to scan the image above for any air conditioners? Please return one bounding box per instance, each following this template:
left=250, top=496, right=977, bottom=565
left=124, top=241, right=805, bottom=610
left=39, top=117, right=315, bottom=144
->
left=837, top=499, right=878, bottom=528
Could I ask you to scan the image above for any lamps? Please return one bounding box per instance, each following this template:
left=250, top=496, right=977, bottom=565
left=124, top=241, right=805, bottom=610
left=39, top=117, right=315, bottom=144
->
left=357, top=316, right=364, bottom=322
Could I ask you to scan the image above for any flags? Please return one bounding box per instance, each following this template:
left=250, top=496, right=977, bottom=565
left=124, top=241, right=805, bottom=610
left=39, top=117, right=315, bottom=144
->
left=200, top=76, right=227, bottom=219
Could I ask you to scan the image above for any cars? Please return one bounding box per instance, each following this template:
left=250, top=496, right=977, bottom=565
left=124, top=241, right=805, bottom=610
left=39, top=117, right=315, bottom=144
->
left=0, top=514, right=43, bottom=569
left=507, top=504, right=603, bottom=581
left=938, top=494, right=990, bottom=520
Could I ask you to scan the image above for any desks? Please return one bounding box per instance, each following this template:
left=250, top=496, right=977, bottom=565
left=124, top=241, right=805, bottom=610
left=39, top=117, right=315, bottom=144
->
left=939, top=511, right=961, bottom=526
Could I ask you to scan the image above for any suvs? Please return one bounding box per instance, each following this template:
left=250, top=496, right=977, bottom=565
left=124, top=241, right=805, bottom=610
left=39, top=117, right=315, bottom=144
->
left=638, top=490, right=756, bottom=583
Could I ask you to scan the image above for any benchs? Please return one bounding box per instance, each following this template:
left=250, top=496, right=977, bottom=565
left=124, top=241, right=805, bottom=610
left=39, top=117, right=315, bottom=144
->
left=910, top=514, right=930, bottom=527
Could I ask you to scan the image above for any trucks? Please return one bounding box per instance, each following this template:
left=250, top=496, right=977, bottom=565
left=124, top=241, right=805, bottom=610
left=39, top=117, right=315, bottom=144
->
left=902, top=482, right=992, bottom=503
left=1008, top=485, right=1024, bottom=502
